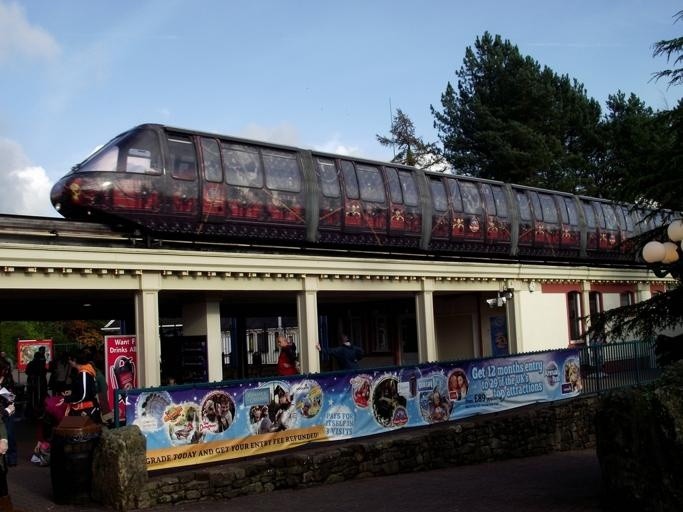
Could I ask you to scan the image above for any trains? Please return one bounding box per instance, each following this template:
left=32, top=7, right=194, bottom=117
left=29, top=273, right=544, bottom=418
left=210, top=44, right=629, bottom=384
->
left=49, top=123, right=683, bottom=269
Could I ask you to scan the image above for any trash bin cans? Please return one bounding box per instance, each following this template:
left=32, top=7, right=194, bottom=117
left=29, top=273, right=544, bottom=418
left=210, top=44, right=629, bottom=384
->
left=49, top=416, right=100, bottom=504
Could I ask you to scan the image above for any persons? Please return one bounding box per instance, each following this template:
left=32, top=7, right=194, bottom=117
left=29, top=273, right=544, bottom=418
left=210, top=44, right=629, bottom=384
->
left=202, top=400, right=218, bottom=433
left=0, top=369, right=14, bottom=511
left=316, top=334, right=364, bottom=370
left=544, top=363, right=558, bottom=385
left=0, top=360, right=17, bottom=468
left=430, top=390, right=450, bottom=417
left=0, top=349, right=14, bottom=372
left=449, top=374, right=459, bottom=400
left=24, top=344, right=107, bottom=466
left=214, top=394, right=232, bottom=430
left=353, top=379, right=373, bottom=406
left=251, top=386, right=291, bottom=434
left=457, top=373, right=467, bottom=401
left=275, top=334, right=299, bottom=375
